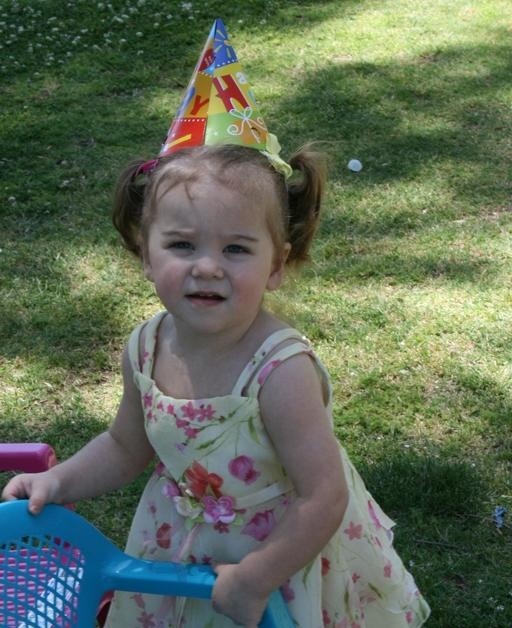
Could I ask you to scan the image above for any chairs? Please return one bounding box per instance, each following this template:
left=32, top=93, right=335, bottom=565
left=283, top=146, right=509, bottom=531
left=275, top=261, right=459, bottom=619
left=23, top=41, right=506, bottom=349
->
left=0, top=499, right=294, bottom=628
left=0, top=442, right=114, bottom=628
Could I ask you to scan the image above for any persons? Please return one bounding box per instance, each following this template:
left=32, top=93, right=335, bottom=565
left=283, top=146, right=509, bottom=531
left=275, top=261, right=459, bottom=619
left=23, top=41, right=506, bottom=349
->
left=2, top=17, right=433, bottom=628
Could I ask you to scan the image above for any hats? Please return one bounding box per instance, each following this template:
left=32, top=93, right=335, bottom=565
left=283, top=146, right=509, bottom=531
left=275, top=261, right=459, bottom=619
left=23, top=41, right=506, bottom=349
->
left=155, top=17, right=276, bottom=158
